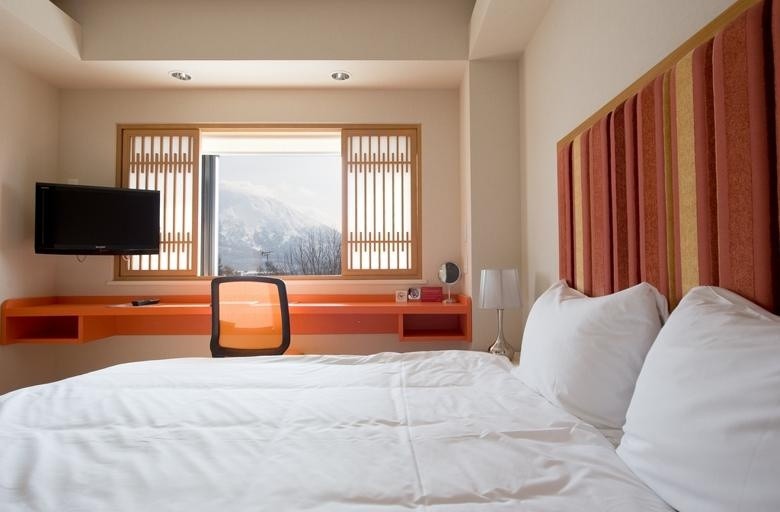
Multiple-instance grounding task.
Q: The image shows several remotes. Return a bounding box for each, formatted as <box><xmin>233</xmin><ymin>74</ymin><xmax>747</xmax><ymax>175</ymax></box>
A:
<box><xmin>132</xmin><ymin>297</ymin><xmax>160</xmax><ymax>306</ymax></box>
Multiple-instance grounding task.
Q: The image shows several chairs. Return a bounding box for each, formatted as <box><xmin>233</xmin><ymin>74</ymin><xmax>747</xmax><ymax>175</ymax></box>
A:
<box><xmin>206</xmin><ymin>275</ymin><xmax>292</xmax><ymax>354</ymax></box>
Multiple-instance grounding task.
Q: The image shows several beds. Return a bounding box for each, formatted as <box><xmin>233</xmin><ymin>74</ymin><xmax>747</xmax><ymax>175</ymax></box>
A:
<box><xmin>1</xmin><ymin>349</ymin><xmax>779</xmax><ymax>512</ymax></box>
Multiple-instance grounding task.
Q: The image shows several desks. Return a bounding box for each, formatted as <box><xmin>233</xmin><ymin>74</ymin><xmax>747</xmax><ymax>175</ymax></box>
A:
<box><xmin>1</xmin><ymin>293</ymin><xmax>471</xmax><ymax>344</ymax></box>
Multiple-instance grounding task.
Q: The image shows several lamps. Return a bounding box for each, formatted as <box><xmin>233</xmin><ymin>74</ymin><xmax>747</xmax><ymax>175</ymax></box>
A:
<box><xmin>478</xmin><ymin>266</ymin><xmax>526</xmax><ymax>359</ymax></box>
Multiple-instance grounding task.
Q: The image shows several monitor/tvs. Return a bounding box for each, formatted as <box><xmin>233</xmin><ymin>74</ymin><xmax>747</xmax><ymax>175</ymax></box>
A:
<box><xmin>35</xmin><ymin>182</ymin><xmax>160</xmax><ymax>255</ymax></box>
